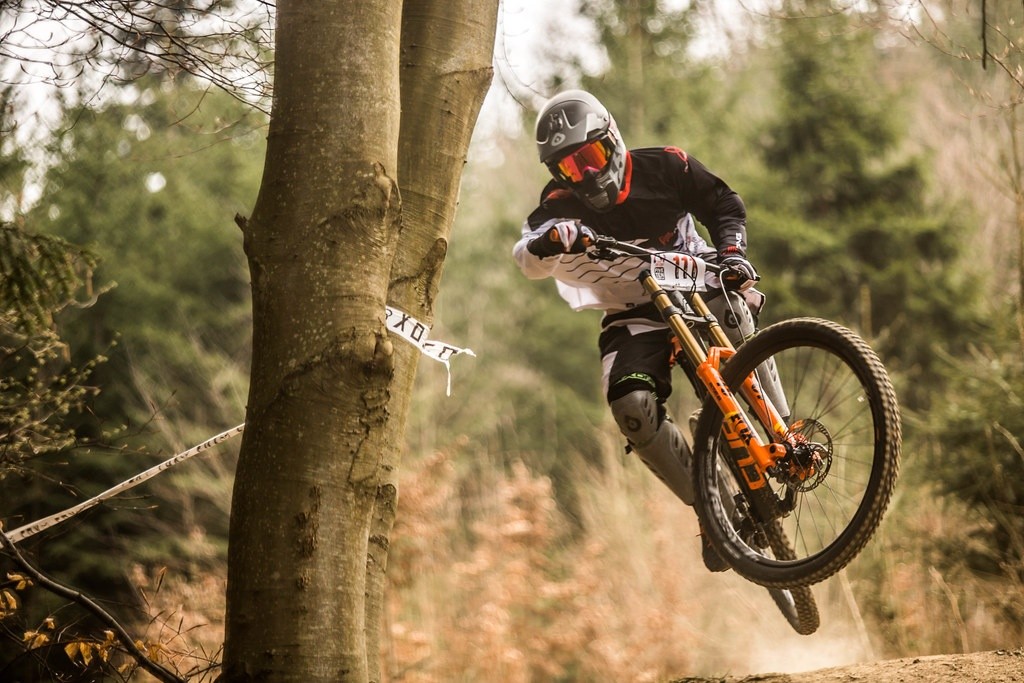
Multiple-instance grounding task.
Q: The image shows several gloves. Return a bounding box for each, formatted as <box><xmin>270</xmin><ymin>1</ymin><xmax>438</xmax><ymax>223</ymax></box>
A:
<box><xmin>717</xmin><ymin>249</ymin><xmax>758</xmax><ymax>292</ymax></box>
<box><xmin>534</xmin><ymin>219</ymin><xmax>598</xmax><ymax>259</ymax></box>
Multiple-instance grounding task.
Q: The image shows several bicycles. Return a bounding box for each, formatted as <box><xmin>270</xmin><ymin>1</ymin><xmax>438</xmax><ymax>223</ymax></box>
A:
<box><xmin>545</xmin><ymin>220</ymin><xmax>901</xmax><ymax>635</ymax></box>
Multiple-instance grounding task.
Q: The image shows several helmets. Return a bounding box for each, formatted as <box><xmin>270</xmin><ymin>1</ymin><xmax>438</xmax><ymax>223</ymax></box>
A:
<box><xmin>534</xmin><ymin>89</ymin><xmax>625</xmax><ymax>213</ymax></box>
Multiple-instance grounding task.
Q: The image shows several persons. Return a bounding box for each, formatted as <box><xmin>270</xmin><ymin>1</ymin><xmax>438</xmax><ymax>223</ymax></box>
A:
<box><xmin>509</xmin><ymin>88</ymin><xmax>802</xmax><ymax>573</ymax></box>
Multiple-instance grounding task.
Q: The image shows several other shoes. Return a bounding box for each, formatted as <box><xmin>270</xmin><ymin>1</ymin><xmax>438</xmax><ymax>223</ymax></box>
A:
<box><xmin>701</xmin><ymin>511</ymin><xmax>732</xmax><ymax>573</ymax></box>
